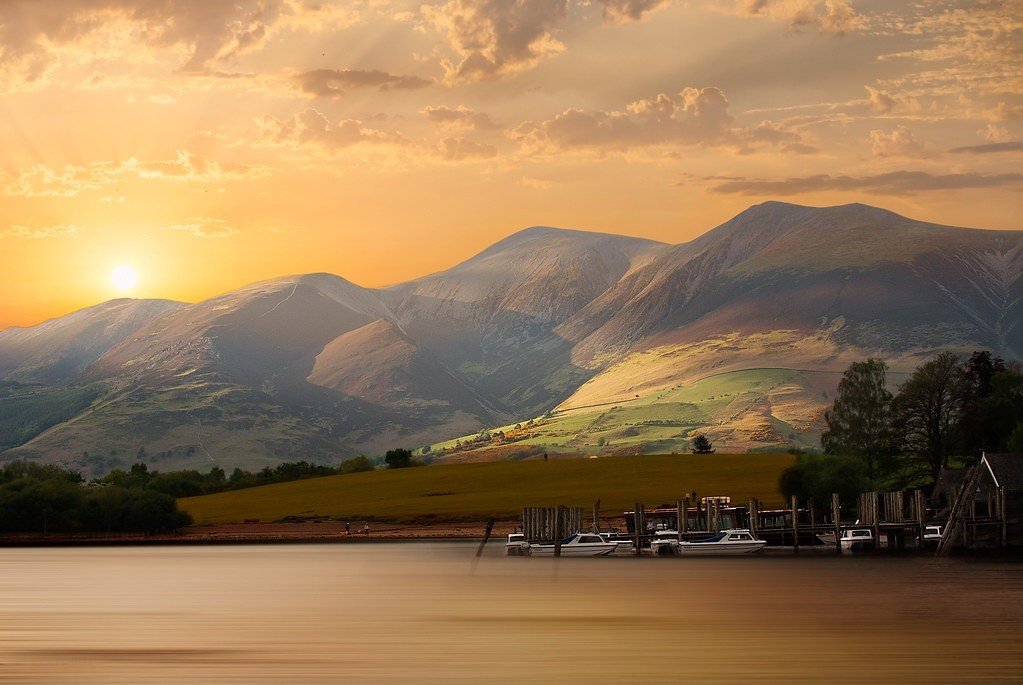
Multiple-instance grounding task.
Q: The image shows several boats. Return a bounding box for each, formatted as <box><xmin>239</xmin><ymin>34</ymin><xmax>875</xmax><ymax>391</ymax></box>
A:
<box><xmin>669</xmin><ymin>528</ymin><xmax>769</xmax><ymax>557</ymax></box>
<box><xmin>506</xmin><ymin>531</ymin><xmax>525</xmax><ymax>549</ymax></box>
<box><xmin>816</xmin><ymin>532</ymin><xmax>838</xmax><ymax>545</ymax></box>
<box><xmin>528</xmin><ymin>534</ymin><xmax>619</xmax><ymax>556</ymax></box>
<box><xmin>837</xmin><ymin>530</ymin><xmax>875</xmax><ymax>552</ymax></box>
<box><xmin>915</xmin><ymin>524</ymin><xmax>943</xmax><ymax>542</ymax></box>
